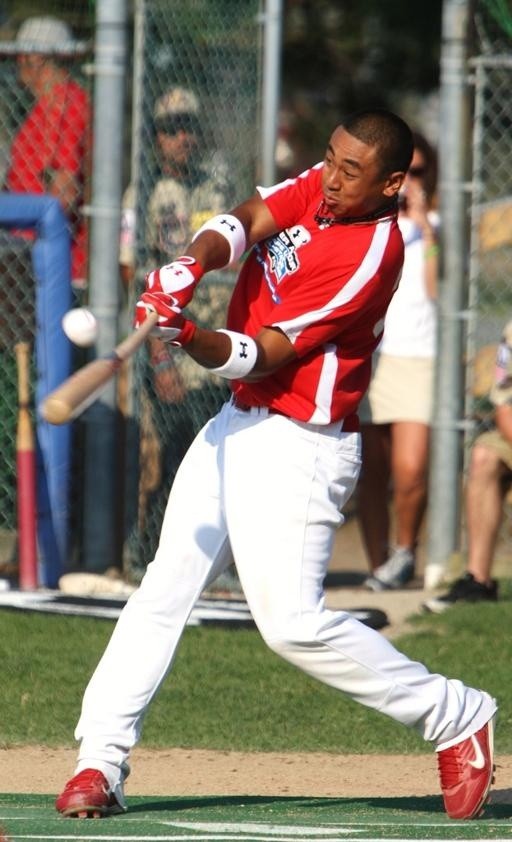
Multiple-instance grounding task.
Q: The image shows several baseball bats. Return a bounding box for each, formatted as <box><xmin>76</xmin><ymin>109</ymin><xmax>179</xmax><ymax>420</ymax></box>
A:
<box><xmin>40</xmin><ymin>311</ymin><xmax>158</xmax><ymax>424</ymax></box>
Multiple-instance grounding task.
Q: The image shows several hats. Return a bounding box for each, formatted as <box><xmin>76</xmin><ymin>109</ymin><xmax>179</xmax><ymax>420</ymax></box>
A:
<box><xmin>15</xmin><ymin>16</ymin><xmax>70</xmax><ymax>42</ymax></box>
<box><xmin>152</xmin><ymin>88</ymin><xmax>203</xmax><ymax>139</ymax></box>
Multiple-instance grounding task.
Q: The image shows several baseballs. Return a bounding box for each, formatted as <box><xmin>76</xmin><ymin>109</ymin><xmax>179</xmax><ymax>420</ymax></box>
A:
<box><xmin>62</xmin><ymin>307</ymin><xmax>98</xmax><ymax>346</ymax></box>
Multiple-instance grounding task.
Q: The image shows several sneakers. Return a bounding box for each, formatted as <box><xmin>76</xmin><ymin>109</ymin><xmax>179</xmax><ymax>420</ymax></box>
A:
<box><xmin>361</xmin><ymin>552</ymin><xmax>501</xmax><ymax>616</ymax></box>
<box><xmin>56</xmin><ymin>768</ymin><xmax>118</xmax><ymax>818</ymax></box>
<box><xmin>438</xmin><ymin>717</ymin><xmax>496</xmax><ymax>820</ymax></box>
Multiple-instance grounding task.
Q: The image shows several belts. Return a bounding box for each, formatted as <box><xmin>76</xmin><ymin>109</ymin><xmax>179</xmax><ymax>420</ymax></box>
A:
<box><xmin>232</xmin><ymin>399</ymin><xmax>360</xmax><ymax>433</ymax></box>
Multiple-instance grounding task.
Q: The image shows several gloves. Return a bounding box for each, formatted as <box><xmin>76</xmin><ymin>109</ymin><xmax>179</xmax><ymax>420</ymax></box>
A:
<box><xmin>133</xmin><ymin>292</ymin><xmax>196</xmax><ymax>348</ymax></box>
<box><xmin>145</xmin><ymin>256</ymin><xmax>206</xmax><ymax>308</ymax></box>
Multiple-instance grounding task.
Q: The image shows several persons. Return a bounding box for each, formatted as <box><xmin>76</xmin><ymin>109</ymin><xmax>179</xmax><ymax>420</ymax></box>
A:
<box><xmin>353</xmin><ymin>130</ymin><xmax>442</xmax><ymax>593</ymax></box>
<box><xmin>56</xmin><ymin>108</ymin><xmax>498</xmax><ymax>820</ymax></box>
<box><xmin>0</xmin><ymin>16</ymin><xmax>93</xmax><ymax>584</ymax></box>
<box><xmin>117</xmin><ymin>87</ymin><xmax>236</xmax><ymax>585</ymax></box>
<box><xmin>418</xmin><ymin>320</ymin><xmax>512</xmax><ymax>615</ymax></box>
